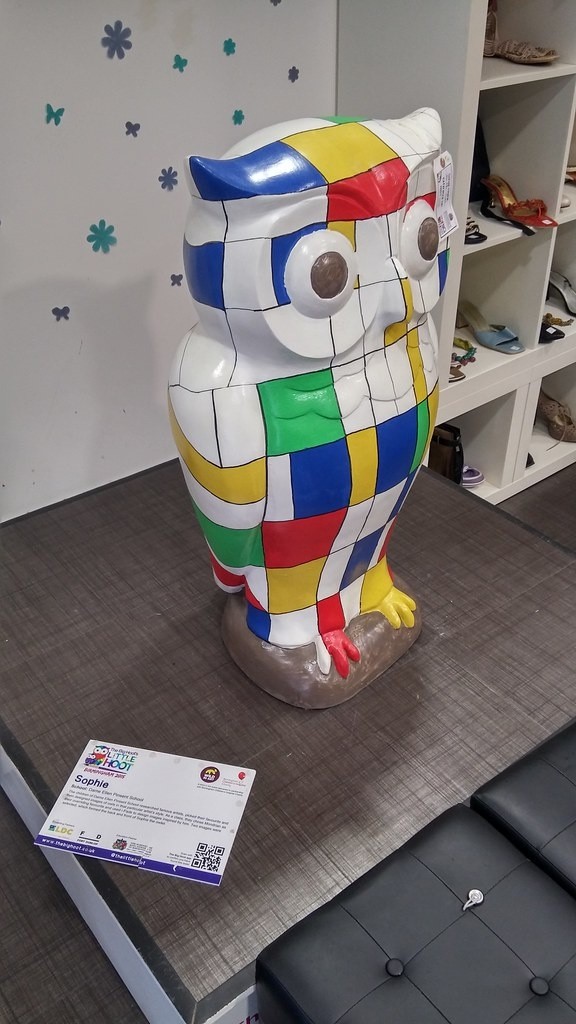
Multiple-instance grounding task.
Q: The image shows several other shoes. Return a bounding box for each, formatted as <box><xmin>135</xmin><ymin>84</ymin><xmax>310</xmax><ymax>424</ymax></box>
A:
<box><xmin>465</xmin><ymin>216</ymin><xmax>487</xmax><ymax>243</ymax></box>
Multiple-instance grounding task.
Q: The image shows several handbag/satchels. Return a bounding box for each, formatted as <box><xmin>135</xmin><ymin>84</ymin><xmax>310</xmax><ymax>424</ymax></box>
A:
<box><xmin>428</xmin><ymin>424</ymin><xmax>464</xmax><ymax>485</ymax></box>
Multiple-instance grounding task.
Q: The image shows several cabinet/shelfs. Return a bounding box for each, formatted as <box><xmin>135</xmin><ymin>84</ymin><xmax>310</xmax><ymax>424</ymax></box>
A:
<box><xmin>335</xmin><ymin>0</ymin><xmax>575</xmax><ymax>506</ymax></box>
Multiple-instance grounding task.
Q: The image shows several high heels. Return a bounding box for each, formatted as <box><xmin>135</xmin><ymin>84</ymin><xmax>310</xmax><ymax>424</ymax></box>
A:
<box><xmin>458</xmin><ymin>299</ymin><xmax>524</xmax><ymax>355</ymax></box>
<box><xmin>546</xmin><ymin>270</ymin><xmax>576</xmax><ymax>316</ymax></box>
<box><xmin>534</xmin><ymin>389</ymin><xmax>576</xmax><ymax>444</ymax></box>
<box><xmin>480</xmin><ymin>175</ymin><xmax>559</xmax><ymax>227</ymax></box>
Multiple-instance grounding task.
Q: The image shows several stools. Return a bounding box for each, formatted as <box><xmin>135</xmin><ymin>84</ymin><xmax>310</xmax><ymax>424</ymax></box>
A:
<box><xmin>255</xmin><ymin>804</ymin><xmax>576</xmax><ymax>1024</ymax></box>
<box><xmin>469</xmin><ymin>722</ymin><xmax>576</xmax><ymax>899</ymax></box>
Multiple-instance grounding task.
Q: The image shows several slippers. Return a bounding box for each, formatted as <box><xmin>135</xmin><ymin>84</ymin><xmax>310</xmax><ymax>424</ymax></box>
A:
<box><xmin>482</xmin><ymin>4</ymin><xmax>497</xmax><ymax>56</ymax></box>
<box><xmin>493</xmin><ymin>36</ymin><xmax>559</xmax><ymax>62</ymax></box>
